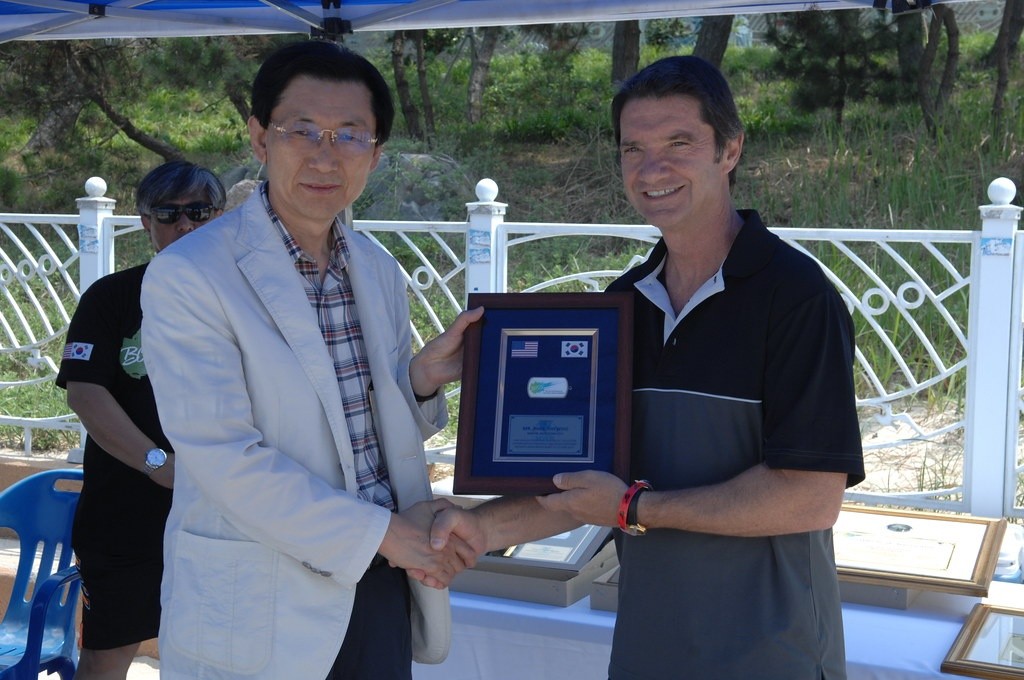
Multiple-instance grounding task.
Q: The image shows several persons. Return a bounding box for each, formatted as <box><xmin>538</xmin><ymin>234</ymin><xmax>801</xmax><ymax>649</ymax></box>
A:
<box><xmin>56</xmin><ymin>163</ymin><xmax>225</xmax><ymax>680</ymax></box>
<box><xmin>140</xmin><ymin>39</ymin><xmax>482</xmax><ymax>680</ymax></box>
<box><xmin>392</xmin><ymin>61</ymin><xmax>868</xmax><ymax>680</ymax></box>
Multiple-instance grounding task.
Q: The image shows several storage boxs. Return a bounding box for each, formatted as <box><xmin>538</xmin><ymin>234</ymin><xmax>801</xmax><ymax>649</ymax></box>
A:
<box><xmin>590</xmin><ymin>564</ymin><xmax>622</xmax><ymax>613</ymax></box>
<box><xmin>448</xmin><ymin>540</ymin><xmax>619</xmax><ymax>608</ymax></box>
<box><xmin>839</xmin><ymin>574</ymin><xmax>924</xmax><ymax>610</ymax></box>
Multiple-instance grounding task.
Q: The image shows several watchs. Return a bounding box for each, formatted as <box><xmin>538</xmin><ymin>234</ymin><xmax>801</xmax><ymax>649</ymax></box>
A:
<box><xmin>626</xmin><ymin>485</ymin><xmax>649</xmax><ymax>538</ymax></box>
<box><xmin>144</xmin><ymin>449</ymin><xmax>168</xmax><ymax>476</ymax></box>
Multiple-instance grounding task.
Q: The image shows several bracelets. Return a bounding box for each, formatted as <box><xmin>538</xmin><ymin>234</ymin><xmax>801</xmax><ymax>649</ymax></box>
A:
<box><xmin>416</xmin><ymin>389</ymin><xmax>442</xmax><ymax>404</ymax></box>
<box><xmin>616</xmin><ymin>480</ymin><xmax>650</xmax><ymax>533</ymax></box>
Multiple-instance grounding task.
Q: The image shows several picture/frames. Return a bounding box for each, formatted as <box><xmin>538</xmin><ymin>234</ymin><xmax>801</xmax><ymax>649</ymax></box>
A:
<box><xmin>453</xmin><ymin>291</ymin><xmax>633</xmax><ymax>497</ymax></box>
<box><xmin>479</xmin><ymin>522</ymin><xmax>612</xmax><ymax>570</ymax></box>
<box><xmin>832</xmin><ymin>503</ymin><xmax>1009</xmax><ymax>598</ymax></box>
<box><xmin>939</xmin><ymin>602</ymin><xmax>1024</xmax><ymax>680</ymax></box>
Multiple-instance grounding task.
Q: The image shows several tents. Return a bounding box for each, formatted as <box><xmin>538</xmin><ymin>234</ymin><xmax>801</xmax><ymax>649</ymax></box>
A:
<box><xmin>0</xmin><ymin>0</ymin><xmax>977</xmax><ymax>43</ymax></box>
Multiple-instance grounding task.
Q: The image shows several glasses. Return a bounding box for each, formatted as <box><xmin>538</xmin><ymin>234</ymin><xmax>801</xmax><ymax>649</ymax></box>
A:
<box><xmin>267</xmin><ymin>120</ymin><xmax>380</xmax><ymax>157</ymax></box>
<box><xmin>152</xmin><ymin>203</ymin><xmax>213</xmax><ymax>225</ymax></box>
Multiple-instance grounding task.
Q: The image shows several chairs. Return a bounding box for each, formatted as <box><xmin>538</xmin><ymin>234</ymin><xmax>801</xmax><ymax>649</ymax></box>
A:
<box><xmin>0</xmin><ymin>469</ymin><xmax>84</xmax><ymax>680</ymax></box>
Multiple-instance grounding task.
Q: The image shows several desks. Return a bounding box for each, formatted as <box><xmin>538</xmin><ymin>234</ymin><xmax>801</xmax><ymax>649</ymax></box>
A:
<box><xmin>411</xmin><ymin>581</ymin><xmax>1024</xmax><ymax>680</ymax></box>
